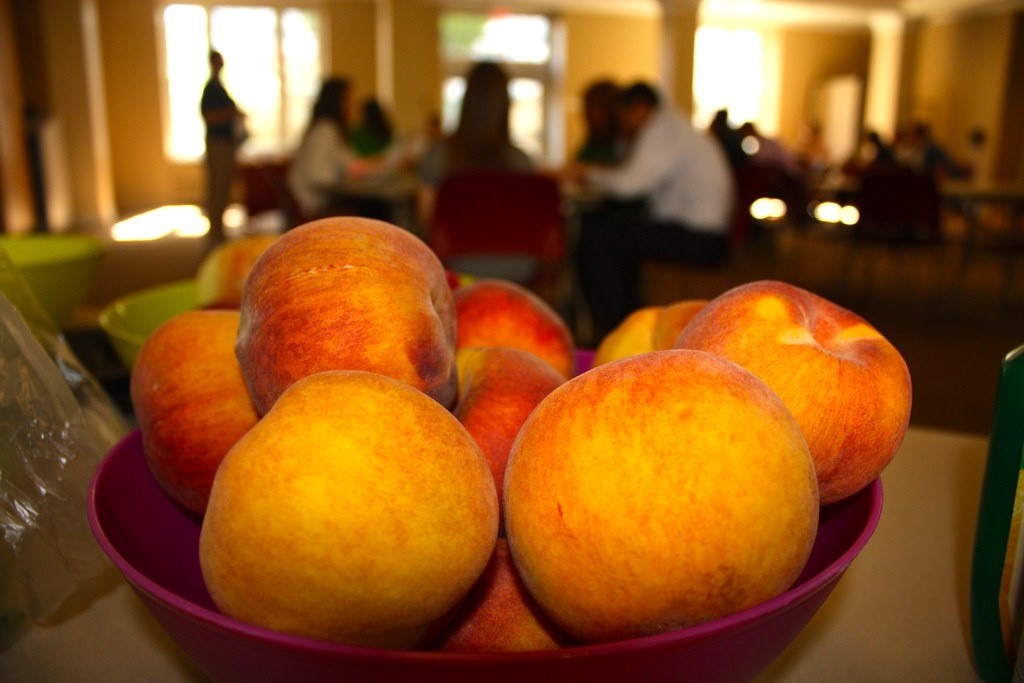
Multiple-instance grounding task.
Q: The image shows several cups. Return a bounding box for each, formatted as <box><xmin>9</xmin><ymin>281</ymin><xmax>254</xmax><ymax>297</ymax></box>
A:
<box><xmin>0</xmin><ymin>242</ymin><xmax>128</xmax><ymax>627</ymax></box>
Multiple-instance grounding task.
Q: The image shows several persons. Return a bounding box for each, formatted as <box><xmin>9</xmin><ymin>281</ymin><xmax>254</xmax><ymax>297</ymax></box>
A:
<box><xmin>413</xmin><ymin>62</ymin><xmax>562</xmax><ymax>299</ymax></box>
<box><xmin>568</xmin><ymin>82</ymin><xmax>738</xmax><ymax>344</ymax></box>
<box><xmin>198</xmin><ymin>52</ymin><xmax>248</xmax><ymax>246</ymax></box>
<box><xmin>541</xmin><ymin>80</ymin><xmax>651</xmax><ymax>246</ymax></box>
<box><xmin>700</xmin><ymin>105</ymin><xmax>980</xmax><ymax>253</ymax></box>
<box><xmin>347</xmin><ymin>93</ymin><xmax>409</xmax><ymax>218</ymax></box>
<box><xmin>282</xmin><ymin>75</ymin><xmax>433</xmax><ymax>219</ymax></box>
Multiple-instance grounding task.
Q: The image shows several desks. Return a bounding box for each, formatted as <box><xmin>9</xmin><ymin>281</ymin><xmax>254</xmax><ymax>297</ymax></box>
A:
<box><xmin>2</xmin><ymin>424</ymin><xmax>990</xmax><ymax>682</ymax></box>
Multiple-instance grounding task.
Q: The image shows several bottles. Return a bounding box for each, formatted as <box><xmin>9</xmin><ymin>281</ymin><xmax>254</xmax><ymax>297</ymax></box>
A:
<box><xmin>969</xmin><ymin>342</ymin><xmax>1024</xmax><ymax>683</ymax></box>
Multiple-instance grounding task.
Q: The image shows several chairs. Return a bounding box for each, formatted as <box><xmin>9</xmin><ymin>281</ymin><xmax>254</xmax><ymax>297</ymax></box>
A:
<box><xmin>428</xmin><ymin>170</ymin><xmax>568</xmax><ymax>327</ymax></box>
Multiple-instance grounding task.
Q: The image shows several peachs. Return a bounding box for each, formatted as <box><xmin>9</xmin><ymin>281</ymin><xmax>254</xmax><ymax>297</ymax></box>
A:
<box><xmin>130</xmin><ymin>216</ymin><xmax>913</xmax><ymax>654</ymax></box>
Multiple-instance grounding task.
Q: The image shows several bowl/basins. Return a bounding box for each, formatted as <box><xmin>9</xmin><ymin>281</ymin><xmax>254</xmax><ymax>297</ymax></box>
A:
<box><xmin>0</xmin><ymin>233</ymin><xmax>106</xmax><ymax>330</ymax></box>
<box><xmin>96</xmin><ymin>280</ymin><xmax>197</xmax><ymax>371</ymax></box>
<box><xmin>88</xmin><ymin>347</ymin><xmax>884</xmax><ymax>683</ymax></box>
